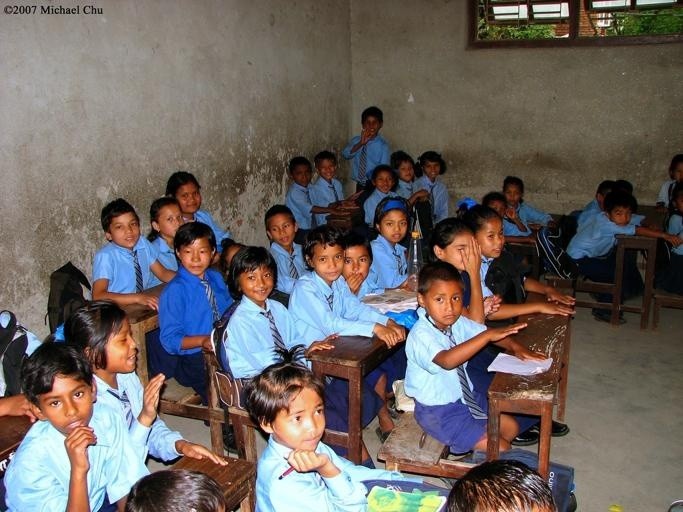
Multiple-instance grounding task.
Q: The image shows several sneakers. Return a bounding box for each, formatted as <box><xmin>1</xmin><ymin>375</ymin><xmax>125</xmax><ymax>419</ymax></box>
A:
<box><xmin>386</xmin><ymin>396</ymin><xmax>405</xmax><ymax>415</ymax></box>
<box><xmin>220</xmin><ymin>424</ymin><xmax>239</xmax><ymax>454</ymax></box>
<box><xmin>375</xmin><ymin>427</ymin><xmax>392</xmax><ymax>445</ymax></box>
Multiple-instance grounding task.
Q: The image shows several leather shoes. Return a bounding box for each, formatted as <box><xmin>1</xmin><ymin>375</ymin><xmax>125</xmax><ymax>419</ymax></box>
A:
<box><xmin>531</xmin><ymin>418</ymin><xmax>571</xmax><ymax>438</ymax></box>
<box><xmin>591</xmin><ymin>307</ymin><xmax>627</xmax><ymax>325</ymax></box>
<box><xmin>511</xmin><ymin>431</ymin><xmax>540</xmax><ymax>446</ymax></box>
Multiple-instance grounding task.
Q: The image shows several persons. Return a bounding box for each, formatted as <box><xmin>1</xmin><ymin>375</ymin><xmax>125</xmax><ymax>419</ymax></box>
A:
<box><xmin>0</xmin><ymin>106</ymin><xmax>683</xmax><ymax>512</ymax></box>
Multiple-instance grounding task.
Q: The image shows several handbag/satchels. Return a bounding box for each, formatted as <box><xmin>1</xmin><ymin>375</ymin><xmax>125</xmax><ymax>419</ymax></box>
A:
<box><xmin>472</xmin><ymin>448</ymin><xmax>575</xmax><ymax>512</ymax></box>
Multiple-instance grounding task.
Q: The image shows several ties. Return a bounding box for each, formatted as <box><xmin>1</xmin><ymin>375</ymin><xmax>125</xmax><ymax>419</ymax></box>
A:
<box><xmin>199</xmin><ymin>279</ymin><xmax>221</xmax><ymax>323</ymax></box>
<box><xmin>406</xmin><ymin>184</ymin><xmax>414</xmax><ymax>196</ymax></box>
<box><xmin>428</xmin><ymin>184</ymin><xmax>436</xmax><ymax>219</ymax></box>
<box><xmin>441</xmin><ymin>325</ymin><xmax>489</xmax><ymax>420</ymax></box>
<box><xmin>326</xmin><ymin>294</ymin><xmax>335</xmax><ymax>312</ymax></box>
<box><xmin>301</xmin><ymin>190</ymin><xmax>318</xmax><ymax>229</ymax></box>
<box><xmin>129</xmin><ymin>250</ymin><xmax>143</xmax><ymax>294</ymax></box>
<box><xmin>259</xmin><ymin>310</ymin><xmax>287</xmax><ymax>354</ymax></box>
<box><xmin>286</xmin><ymin>255</ymin><xmax>300</xmax><ymax>280</ymax></box>
<box><xmin>328</xmin><ymin>184</ymin><xmax>339</xmax><ymax>201</ymax></box>
<box><xmin>393</xmin><ymin>253</ymin><xmax>404</xmax><ymax>277</ymax></box>
<box><xmin>357</xmin><ymin>144</ymin><xmax>367</xmax><ymax>181</ymax></box>
<box><xmin>107</xmin><ymin>390</ymin><xmax>136</xmax><ymax>435</ymax></box>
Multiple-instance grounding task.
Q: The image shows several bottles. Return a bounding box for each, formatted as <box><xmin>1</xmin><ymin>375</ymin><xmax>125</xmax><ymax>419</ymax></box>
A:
<box><xmin>406</xmin><ymin>231</ymin><xmax>421</xmax><ymax>289</ymax></box>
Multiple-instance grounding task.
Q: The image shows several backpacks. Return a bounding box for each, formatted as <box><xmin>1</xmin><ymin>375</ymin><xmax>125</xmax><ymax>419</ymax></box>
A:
<box><xmin>534</xmin><ymin>225</ymin><xmax>577</xmax><ymax>281</ymax></box>
<box><xmin>485</xmin><ymin>253</ymin><xmax>528</xmax><ymax>325</ymax></box>
<box><xmin>46</xmin><ymin>262</ymin><xmax>90</xmax><ymax>336</ymax></box>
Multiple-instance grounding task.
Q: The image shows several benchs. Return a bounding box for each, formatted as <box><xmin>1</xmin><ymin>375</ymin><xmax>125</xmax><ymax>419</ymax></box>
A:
<box><xmin>230</xmin><ymin>406</ymin><xmax>261</xmax><ymax>459</ymax></box>
<box><xmin>159</xmin><ymin>376</ymin><xmax>209</xmax><ymax>421</ymax></box>
<box><xmin>653</xmin><ymin>290</ymin><xmax>683</xmax><ymax>330</ymax></box>
<box><xmin>377</xmin><ymin>411</ymin><xmax>476</xmax><ymax>477</ymax></box>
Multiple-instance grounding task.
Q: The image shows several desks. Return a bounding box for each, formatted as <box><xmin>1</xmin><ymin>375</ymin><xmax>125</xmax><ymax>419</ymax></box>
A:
<box><xmin>487</xmin><ymin>289</ymin><xmax>573</xmax><ymax>482</ymax></box>
<box><xmin>121</xmin><ymin>281</ymin><xmax>167</xmax><ymax>389</ymax></box>
<box><xmin>203</xmin><ymin>337</ymin><xmax>224</xmax><ymax>455</ymax></box>
<box><xmin>507</xmin><ymin>214</ymin><xmax>560</xmax><ymax>280</ymax></box>
<box><xmin>326</xmin><ymin>210</ymin><xmax>362</xmax><ymax>228</ymax></box>
<box><xmin>611</xmin><ymin>206</ymin><xmax>657</xmax><ymax>330</ymax></box>
<box><xmin>171</xmin><ymin>455</ymin><xmax>257</xmax><ymax>511</ymax></box>
<box><xmin>0</xmin><ymin>416</ymin><xmax>33</xmax><ymax>461</ymax></box>
<box><xmin>309</xmin><ymin>336</ymin><xmax>405</xmax><ymax>466</ymax></box>
